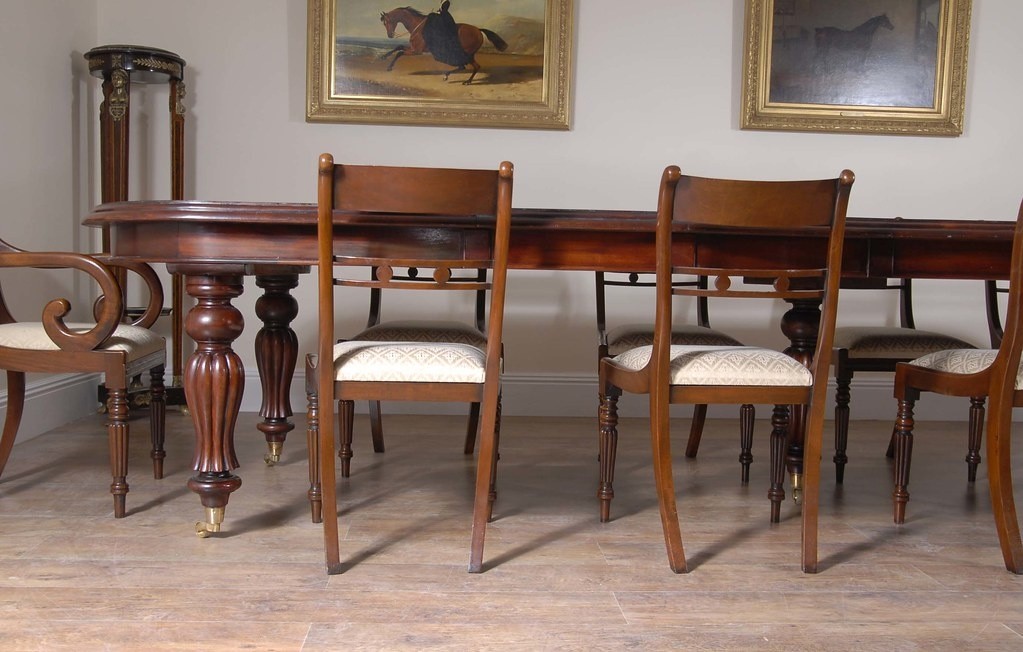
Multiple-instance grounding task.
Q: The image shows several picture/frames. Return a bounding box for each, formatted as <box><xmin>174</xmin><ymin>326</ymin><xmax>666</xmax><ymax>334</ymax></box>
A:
<box><xmin>305</xmin><ymin>0</ymin><xmax>573</xmax><ymax>131</ymax></box>
<box><xmin>739</xmin><ymin>0</ymin><xmax>973</xmax><ymax>136</ymax></box>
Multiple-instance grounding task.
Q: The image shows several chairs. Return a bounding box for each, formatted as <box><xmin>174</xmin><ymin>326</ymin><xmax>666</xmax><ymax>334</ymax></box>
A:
<box><xmin>305</xmin><ymin>152</ymin><xmax>514</xmax><ymax>578</ymax></box>
<box><xmin>597</xmin><ymin>166</ymin><xmax>854</xmax><ymax>576</ymax></box>
<box><xmin>810</xmin><ymin>218</ymin><xmax>987</xmax><ymax>481</ymax></box>
<box><xmin>0</xmin><ymin>238</ymin><xmax>167</xmax><ymax>520</ymax></box>
<box><xmin>893</xmin><ymin>200</ymin><xmax>1023</xmax><ymax>574</ymax></box>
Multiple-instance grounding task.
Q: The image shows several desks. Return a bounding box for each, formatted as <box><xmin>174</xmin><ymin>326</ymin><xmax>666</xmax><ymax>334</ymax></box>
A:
<box><xmin>83</xmin><ymin>200</ymin><xmax>1019</xmax><ymax>541</ymax></box>
